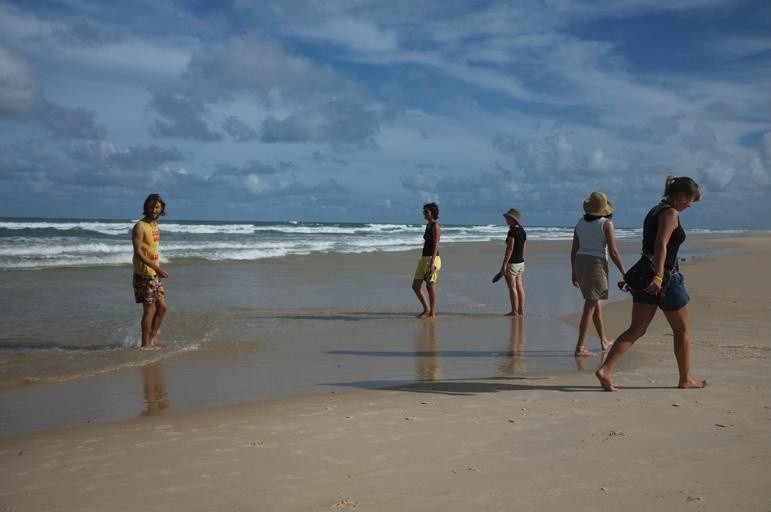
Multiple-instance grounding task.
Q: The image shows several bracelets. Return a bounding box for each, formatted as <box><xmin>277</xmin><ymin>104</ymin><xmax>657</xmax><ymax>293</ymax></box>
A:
<box><xmin>653</xmin><ymin>274</ymin><xmax>663</xmax><ymax>282</ymax></box>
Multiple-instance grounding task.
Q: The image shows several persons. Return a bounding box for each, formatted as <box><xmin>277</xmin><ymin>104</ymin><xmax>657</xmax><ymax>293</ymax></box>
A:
<box><xmin>593</xmin><ymin>173</ymin><xmax>708</xmax><ymax>392</ymax></box>
<box><xmin>411</xmin><ymin>201</ymin><xmax>444</xmax><ymax>320</ymax></box>
<box><xmin>129</xmin><ymin>192</ymin><xmax>170</xmax><ymax>348</ymax></box>
<box><xmin>499</xmin><ymin>208</ymin><xmax>528</xmax><ymax>317</ymax></box>
<box><xmin>569</xmin><ymin>191</ymin><xmax>627</xmax><ymax>357</ymax></box>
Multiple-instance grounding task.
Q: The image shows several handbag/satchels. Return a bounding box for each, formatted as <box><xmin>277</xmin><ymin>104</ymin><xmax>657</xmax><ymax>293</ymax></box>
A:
<box><xmin>623</xmin><ymin>255</ymin><xmax>673</xmax><ymax>302</ymax></box>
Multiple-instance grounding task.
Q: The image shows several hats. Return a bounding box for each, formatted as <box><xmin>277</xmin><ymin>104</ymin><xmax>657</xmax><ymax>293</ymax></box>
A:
<box><xmin>502</xmin><ymin>208</ymin><xmax>520</xmax><ymax>225</ymax></box>
<box><xmin>583</xmin><ymin>192</ymin><xmax>613</xmax><ymax>216</ymax></box>
